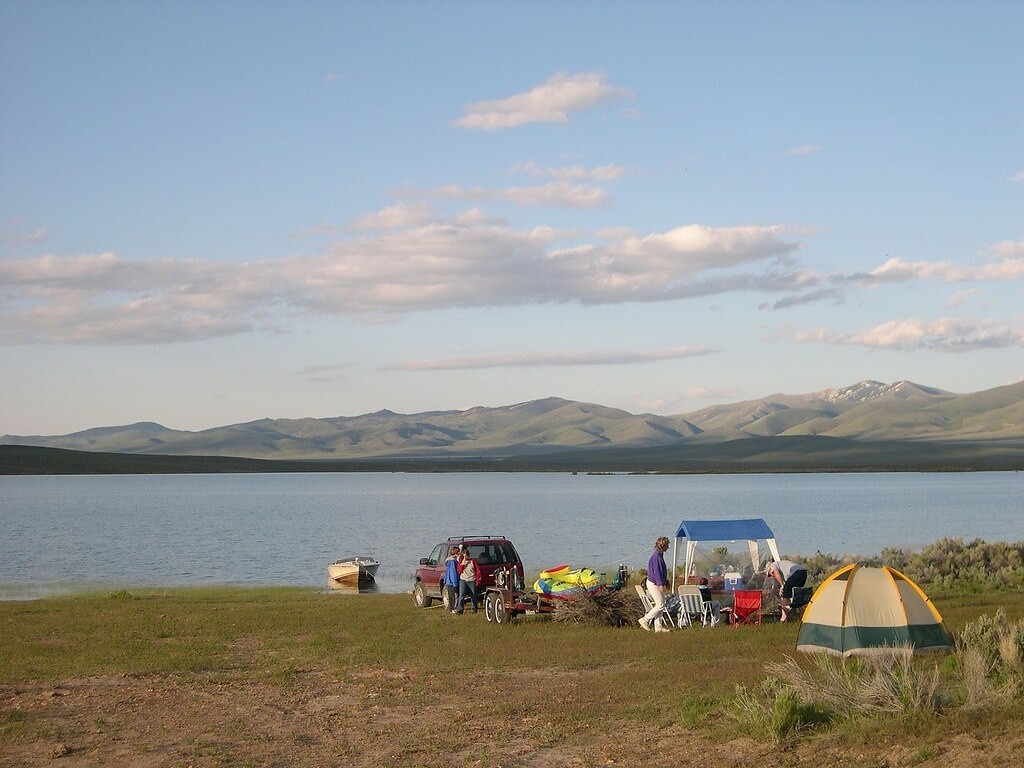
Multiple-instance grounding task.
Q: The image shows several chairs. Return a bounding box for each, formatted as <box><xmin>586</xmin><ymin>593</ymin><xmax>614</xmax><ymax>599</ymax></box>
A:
<box><xmin>731</xmin><ymin>590</ymin><xmax>763</xmax><ymax>628</ymax></box>
<box><xmin>677</xmin><ymin>585</ymin><xmax>716</xmax><ymax>629</ymax></box>
<box><xmin>746</xmin><ymin>570</ymin><xmax>767</xmax><ymax>588</ymax></box>
<box><xmin>478</xmin><ymin>552</ymin><xmax>491</xmax><ymax>561</ymax></box>
<box><xmin>779</xmin><ymin>586</ymin><xmax>813</xmax><ymax>623</ymax></box>
<box><xmin>634</xmin><ymin>585</ymin><xmax>680</xmax><ymax>627</ymax></box>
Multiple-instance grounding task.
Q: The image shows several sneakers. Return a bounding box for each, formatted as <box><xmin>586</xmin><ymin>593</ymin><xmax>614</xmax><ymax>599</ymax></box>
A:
<box><xmin>638</xmin><ymin>618</ymin><xmax>651</xmax><ymax>632</ymax></box>
<box><xmin>452</xmin><ymin>610</ymin><xmax>460</xmax><ymax>616</ymax></box>
<box><xmin>654</xmin><ymin>626</ymin><xmax>670</xmax><ymax>633</ymax></box>
<box><xmin>703</xmin><ymin>621</ymin><xmax>708</xmax><ymax>625</ymax></box>
<box><xmin>711</xmin><ymin>623</ymin><xmax>720</xmax><ymax>627</ymax></box>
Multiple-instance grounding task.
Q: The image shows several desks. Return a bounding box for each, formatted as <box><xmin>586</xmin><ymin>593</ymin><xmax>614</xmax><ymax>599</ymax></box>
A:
<box><xmin>679</xmin><ymin>576</ymin><xmax>723</xmax><ymax>590</ymax></box>
<box><xmin>710</xmin><ymin>589</ymin><xmax>779</xmax><ymax>616</ymax></box>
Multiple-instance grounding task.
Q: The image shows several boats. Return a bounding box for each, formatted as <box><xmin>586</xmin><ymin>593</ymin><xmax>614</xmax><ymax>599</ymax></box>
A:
<box><xmin>532</xmin><ymin>564</ymin><xmax>607</xmax><ymax>601</ymax></box>
<box><xmin>328</xmin><ymin>555</ymin><xmax>381</xmax><ymax>590</ymax></box>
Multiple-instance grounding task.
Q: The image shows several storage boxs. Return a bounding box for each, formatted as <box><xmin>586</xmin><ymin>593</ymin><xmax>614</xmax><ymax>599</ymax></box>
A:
<box><xmin>723</xmin><ymin>573</ymin><xmax>744</xmax><ymax>590</ymax></box>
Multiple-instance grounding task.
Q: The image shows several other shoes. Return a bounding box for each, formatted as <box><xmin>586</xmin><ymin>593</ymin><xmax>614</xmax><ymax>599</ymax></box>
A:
<box><xmin>780</xmin><ymin>616</ymin><xmax>788</xmax><ymax>625</ymax></box>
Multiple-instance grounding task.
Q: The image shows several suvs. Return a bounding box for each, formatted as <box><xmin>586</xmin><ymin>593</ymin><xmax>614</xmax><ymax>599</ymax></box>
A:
<box><xmin>414</xmin><ymin>536</ymin><xmax>525</xmax><ymax>607</ymax></box>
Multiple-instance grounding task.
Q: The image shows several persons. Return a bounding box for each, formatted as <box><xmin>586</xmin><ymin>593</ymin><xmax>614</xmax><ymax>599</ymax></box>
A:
<box><xmin>698</xmin><ymin>578</ymin><xmax>720</xmax><ymax>625</ymax></box>
<box><xmin>637</xmin><ymin>537</ymin><xmax>671</xmax><ymax>632</ymax></box>
<box><xmin>452</xmin><ymin>550</ymin><xmax>481</xmax><ymax>616</ymax></box>
<box><xmin>443</xmin><ymin>547</ymin><xmax>461</xmax><ymax>613</ymax></box>
<box><xmin>764</xmin><ymin>560</ymin><xmax>808</xmax><ymax>623</ymax></box>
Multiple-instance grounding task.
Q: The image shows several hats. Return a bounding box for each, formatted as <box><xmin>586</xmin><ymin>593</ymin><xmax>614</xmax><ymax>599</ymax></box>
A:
<box><xmin>765</xmin><ymin>561</ymin><xmax>772</xmax><ymax>577</ymax></box>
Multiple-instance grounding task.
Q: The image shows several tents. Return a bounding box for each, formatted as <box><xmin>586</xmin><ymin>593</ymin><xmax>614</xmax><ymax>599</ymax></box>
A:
<box><xmin>672</xmin><ymin>519</ymin><xmax>781</xmax><ymax>594</ymax></box>
<box><xmin>795</xmin><ymin>562</ymin><xmax>956</xmax><ymax>659</ymax></box>
